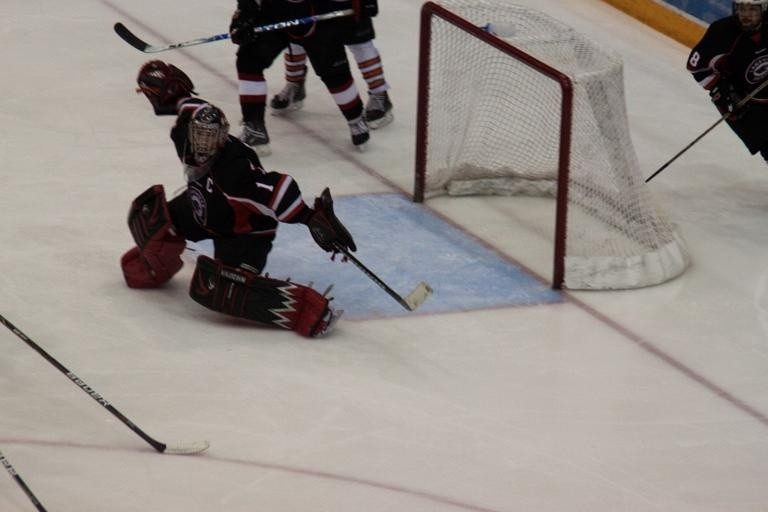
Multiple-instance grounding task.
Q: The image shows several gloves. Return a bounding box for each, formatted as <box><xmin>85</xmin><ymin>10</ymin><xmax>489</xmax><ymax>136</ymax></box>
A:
<box><xmin>230</xmin><ymin>0</ymin><xmax>259</xmax><ymax>45</ymax></box>
<box><xmin>308</xmin><ymin>188</ymin><xmax>356</xmax><ymax>252</ymax></box>
<box><xmin>138</xmin><ymin>60</ymin><xmax>193</xmax><ymax>115</ymax></box>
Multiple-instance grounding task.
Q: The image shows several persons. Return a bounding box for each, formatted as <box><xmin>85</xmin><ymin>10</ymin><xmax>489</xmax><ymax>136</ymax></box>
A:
<box><xmin>685</xmin><ymin>1</ymin><xmax>768</xmax><ymax>159</ymax></box>
<box><xmin>118</xmin><ymin>60</ymin><xmax>358</xmax><ymax>334</ymax></box>
<box><xmin>269</xmin><ymin>1</ymin><xmax>396</xmax><ymax>124</ymax></box>
<box><xmin>229</xmin><ymin>0</ymin><xmax>371</xmax><ymax>153</ymax></box>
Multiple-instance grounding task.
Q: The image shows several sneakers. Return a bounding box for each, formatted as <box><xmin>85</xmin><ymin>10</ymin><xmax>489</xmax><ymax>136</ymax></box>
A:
<box><xmin>271</xmin><ymin>83</ymin><xmax>305</xmax><ymax>108</ymax></box>
<box><xmin>238</xmin><ymin>120</ymin><xmax>268</xmax><ymax>145</ymax></box>
<box><xmin>348</xmin><ymin>91</ymin><xmax>392</xmax><ymax>145</ymax></box>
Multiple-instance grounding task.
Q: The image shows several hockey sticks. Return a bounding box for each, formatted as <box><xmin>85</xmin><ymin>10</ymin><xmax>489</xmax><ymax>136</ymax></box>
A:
<box><xmin>0</xmin><ymin>316</ymin><xmax>208</xmax><ymax>455</ymax></box>
<box><xmin>332</xmin><ymin>240</ymin><xmax>432</xmax><ymax>311</ymax></box>
<box><xmin>115</xmin><ymin>9</ymin><xmax>355</xmax><ymax>53</ymax></box>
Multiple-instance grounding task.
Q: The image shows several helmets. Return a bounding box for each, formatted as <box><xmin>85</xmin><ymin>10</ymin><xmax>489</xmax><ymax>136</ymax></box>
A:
<box><xmin>189</xmin><ymin>103</ymin><xmax>230</xmax><ymax>150</ymax></box>
